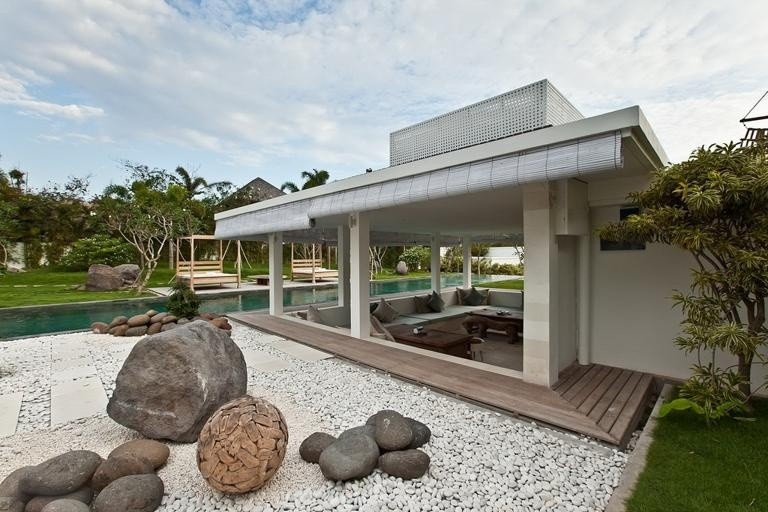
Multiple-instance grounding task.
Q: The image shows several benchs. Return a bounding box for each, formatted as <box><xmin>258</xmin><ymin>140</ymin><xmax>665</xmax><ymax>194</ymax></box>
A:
<box><xmin>383</xmin><ymin>287</ymin><xmax>470</xmax><ymax>327</ymax></box>
<box><xmin>177</xmin><ymin>260</ymin><xmax>240</xmax><ymax>293</ymax></box>
<box><xmin>292</xmin><ymin>259</ymin><xmax>339</xmax><ymax>283</ymax></box>
<box><xmin>470</xmin><ymin>288</ymin><xmax>523</xmax><ymax>312</ymax></box>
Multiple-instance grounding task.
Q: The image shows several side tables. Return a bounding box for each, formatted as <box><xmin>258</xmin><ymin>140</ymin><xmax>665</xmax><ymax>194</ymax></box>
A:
<box><xmin>247</xmin><ymin>274</ymin><xmax>288</xmax><ymax>286</ymax></box>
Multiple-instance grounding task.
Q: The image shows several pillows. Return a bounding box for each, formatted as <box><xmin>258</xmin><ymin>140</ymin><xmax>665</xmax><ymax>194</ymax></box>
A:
<box><xmin>369</xmin><ymin>296</ymin><xmax>398</xmax><ymax>324</ymax></box>
<box><xmin>292</xmin><ymin>304</ymin><xmax>327</xmax><ymax>324</ymax></box>
